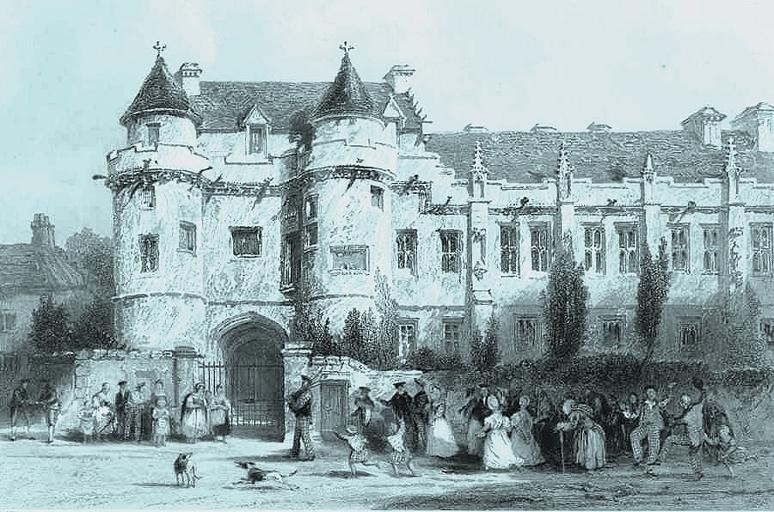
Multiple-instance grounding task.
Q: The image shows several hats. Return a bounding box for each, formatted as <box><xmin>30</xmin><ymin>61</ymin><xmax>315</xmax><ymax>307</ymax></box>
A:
<box><xmin>393</xmin><ymin>382</ymin><xmax>406</xmax><ymax>388</ymax></box>
<box><xmin>414</xmin><ymin>378</ymin><xmax>426</xmax><ymax>386</ymax></box>
<box><xmin>301</xmin><ymin>375</ymin><xmax>312</xmax><ymax>382</ymax></box>
<box><xmin>118</xmin><ymin>381</ymin><xmax>127</xmax><ymax>385</ymax></box>
<box><xmin>359</xmin><ymin>387</ymin><xmax>371</xmax><ymax>392</ymax></box>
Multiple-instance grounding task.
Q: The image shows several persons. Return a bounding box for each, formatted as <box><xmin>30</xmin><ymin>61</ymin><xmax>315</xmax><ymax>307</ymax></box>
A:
<box><xmin>8</xmin><ymin>379</ymin><xmax>39</xmax><ymax>440</ymax></box>
<box><xmin>79</xmin><ymin>381</ymin><xmax>232</xmax><ymax>447</ymax></box>
<box><xmin>282</xmin><ymin>375</ymin><xmax>315</xmax><ymax>461</ymax></box>
<box><xmin>34</xmin><ymin>385</ymin><xmax>63</xmax><ymax>443</ymax></box>
<box><xmin>332</xmin><ymin>377</ymin><xmax>737</xmax><ymax>480</ymax></box>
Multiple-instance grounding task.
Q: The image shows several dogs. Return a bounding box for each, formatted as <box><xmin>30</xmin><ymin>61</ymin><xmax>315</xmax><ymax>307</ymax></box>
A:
<box><xmin>231</xmin><ymin>460</ymin><xmax>299</xmax><ymax>491</ymax></box>
<box><xmin>173</xmin><ymin>452</ymin><xmax>199</xmax><ymax>487</ymax></box>
